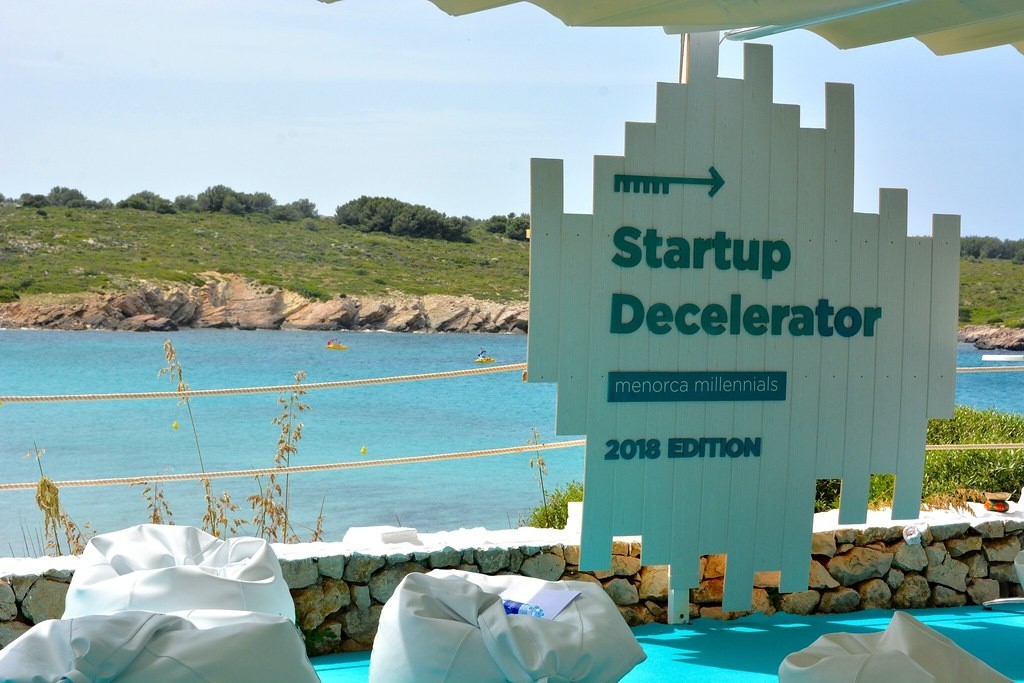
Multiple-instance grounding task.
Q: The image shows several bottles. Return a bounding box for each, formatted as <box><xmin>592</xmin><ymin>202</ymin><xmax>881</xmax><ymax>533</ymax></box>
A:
<box><xmin>502</xmin><ymin>599</ymin><xmax>544</xmax><ymax>618</ymax></box>
